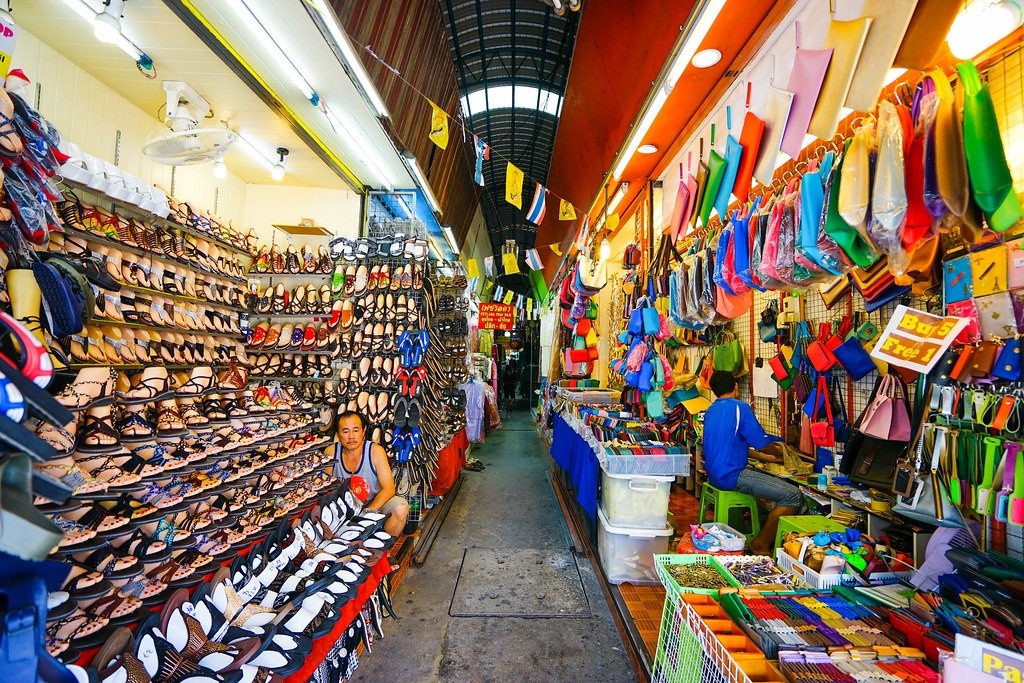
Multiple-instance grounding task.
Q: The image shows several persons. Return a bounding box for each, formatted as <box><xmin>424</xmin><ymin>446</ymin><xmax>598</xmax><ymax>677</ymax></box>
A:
<box><xmin>323</xmin><ymin>410</ymin><xmax>409</xmax><ymax>537</ymax></box>
<box><xmin>502</xmin><ymin>358</ymin><xmax>519</xmax><ymax>408</ymax></box>
<box><xmin>703</xmin><ymin>370</ymin><xmax>804</xmax><ymax>557</ymax></box>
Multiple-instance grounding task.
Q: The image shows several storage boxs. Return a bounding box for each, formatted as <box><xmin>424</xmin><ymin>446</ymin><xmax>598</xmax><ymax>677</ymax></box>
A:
<box><xmin>556</xmin><ymin>386</ymin><xmax>622</xmax><ymax>404</ymax></box>
<box><xmin>595</xmin><ymin>503</ymin><xmax>674</xmax><ymax>585</ymax></box>
<box><xmin>598</xmin><ymin>463</ymin><xmax>677</xmax><ymax>529</ymax></box>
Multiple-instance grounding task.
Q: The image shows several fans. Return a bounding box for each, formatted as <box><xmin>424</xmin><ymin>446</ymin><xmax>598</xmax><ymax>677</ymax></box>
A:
<box><xmin>143</xmin><ymin>81</ymin><xmax>234</xmax><ymax>161</ymax></box>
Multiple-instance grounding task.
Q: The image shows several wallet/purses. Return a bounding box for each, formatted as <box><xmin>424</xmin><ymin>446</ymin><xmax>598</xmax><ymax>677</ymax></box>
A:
<box><xmin>722</xmin><ymin>546</ymin><xmax>1024</xmax><ymax>683</ymax></box>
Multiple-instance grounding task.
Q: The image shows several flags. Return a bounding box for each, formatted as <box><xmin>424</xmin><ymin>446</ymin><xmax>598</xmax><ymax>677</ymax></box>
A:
<box><xmin>470</xmin><ymin>276</ymin><xmax>480</xmax><ymax>292</ymax></box>
<box><xmin>484</xmin><ymin>256</ymin><xmax>494</xmax><ymax>277</ymax></box>
<box><xmin>493</xmin><ymin>285</ymin><xmax>503</xmax><ymax>302</ymax></box>
<box><xmin>503</xmin><ymin>290</ymin><xmax>514</xmax><ymax>305</ymax></box>
<box><xmin>549</xmin><ymin>242</ymin><xmax>563</xmax><ymax>257</ymax></box>
<box><xmin>526</xmin><ymin>182</ymin><xmax>551</xmax><ymax>226</ymax></box>
<box><xmin>515</xmin><ymin>293</ymin><xmax>524</xmax><ymax>309</ymax></box>
<box><xmin>426</xmin><ymin>98</ymin><xmax>451</xmax><ymax>151</ymax></box>
<box><xmin>558</xmin><ymin>198</ymin><xmax>577</xmax><ymax>220</ymax></box>
<box><xmin>474</xmin><ymin>135</ymin><xmax>490</xmax><ymax>187</ymax></box>
<box><xmin>503</xmin><ymin>252</ymin><xmax>520</xmax><ymax>275</ymax></box>
<box><xmin>485</xmin><ymin>281</ymin><xmax>548</xmax><ymax>328</ymax></box>
<box><xmin>524</xmin><ymin>248</ymin><xmax>545</xmax><ymax>271</ymax></box>
<box><xmin>505</xmin><ymin>161</ymin><xmax>524</xmax><ymax>210</ymax></box>
<box><xmin>573</xmin><ymin>213</ymin><xmax>591</xmax><ymax>254</ymax></box>
<box><xmin>466</xmin><ymin>259</ymin><xmax>481</xmax><ymax>278</ymax></box>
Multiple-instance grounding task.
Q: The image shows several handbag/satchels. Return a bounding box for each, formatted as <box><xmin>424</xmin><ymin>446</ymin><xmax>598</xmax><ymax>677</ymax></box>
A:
<box><xmin>558</xmin><ymin>0</ymin><xmax>1023</xmax><ymax>535</ymax></box>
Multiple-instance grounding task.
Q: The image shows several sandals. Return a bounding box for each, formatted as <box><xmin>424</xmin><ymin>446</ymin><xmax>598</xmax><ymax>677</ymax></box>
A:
<box><xmin>0</xmin><ymin>84</ymin><xmax>471</xmax><ymax>683</ymax></box>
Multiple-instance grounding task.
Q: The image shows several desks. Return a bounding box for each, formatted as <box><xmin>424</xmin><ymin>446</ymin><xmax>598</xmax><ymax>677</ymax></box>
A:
<box><xmin>551</xmin><ymin>409</ymin><xmax>686</xmax><ymax>544</ymax></box>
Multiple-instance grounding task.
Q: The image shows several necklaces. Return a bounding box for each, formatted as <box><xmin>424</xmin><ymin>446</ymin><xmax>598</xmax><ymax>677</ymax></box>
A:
<box><xmin>347</xmin><ymin>463</ymin><xmax>357</xmax><ymax>476</ymax></box>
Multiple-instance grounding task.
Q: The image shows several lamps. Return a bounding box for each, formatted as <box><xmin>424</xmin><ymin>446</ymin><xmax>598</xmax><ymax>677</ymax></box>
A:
<box><xmin>212</xmin><ymin>151</ymin><xmax>227</xmax><ymax>179</ymax></box>
<box><xmin>271</xmin><ymin>148</ymin><xmax>289</xmax><ymax>180</ymax></box>
<box><xmin>229</xmin><ymin>0</ymin><xmax>460</xmax><ymax>254</ymax></box>
<box><xmin>68</xmin><ymin>0</ymin><xmax>154</xmax><ymax>68</ymax></box>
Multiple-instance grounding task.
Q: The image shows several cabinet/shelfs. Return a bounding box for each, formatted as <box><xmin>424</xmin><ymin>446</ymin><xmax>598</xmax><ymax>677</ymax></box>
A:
<box><xmin>52</xmin><ymin>172</ymin><xmax>396</xmax><ymax>683</ymax></box>
<box><xmin>748</xmin><ymin>457</ymin><xmax>935</xmax><ymax>572</ymax></box>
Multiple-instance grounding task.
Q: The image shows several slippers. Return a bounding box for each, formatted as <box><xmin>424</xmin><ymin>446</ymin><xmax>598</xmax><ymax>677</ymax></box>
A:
<box><xmin>464</xmin><ymin>458</ymin><xmax>485</xmax><ymax>472</ymax></box>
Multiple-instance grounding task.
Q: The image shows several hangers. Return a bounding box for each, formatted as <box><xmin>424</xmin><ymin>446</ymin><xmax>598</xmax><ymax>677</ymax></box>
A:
<box><xmin>466</xmin><ymin>376</ymin><xmax>475</xmax><ymax>384</ymax></box>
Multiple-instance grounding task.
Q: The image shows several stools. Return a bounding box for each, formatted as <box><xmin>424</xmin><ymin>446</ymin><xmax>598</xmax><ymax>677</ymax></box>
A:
<box><xmin>699</xmin><ymin>482</ymin><xmax>760</xmax><ymax>551</ymax></box>
<box><xmin>773</xmin><ymin>514</ymin><xmax>848</xmax><ymax>562</ymax></box>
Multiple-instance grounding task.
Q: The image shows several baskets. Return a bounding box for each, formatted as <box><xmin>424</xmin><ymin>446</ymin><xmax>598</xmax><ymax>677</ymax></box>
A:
<box><xmin>774</xmin><ymin>537</ymin><xmax>920</xmax><ymax>590</ymax></box>
<box><xmin>709</xmin><ymin>553</ymin><xmax>812</xmax><ymax>594</ymax></box>
<box><xmin>654</xmin><ymin>552</ymin><xmax>743</xmax><ymax>602</ymax></box>
